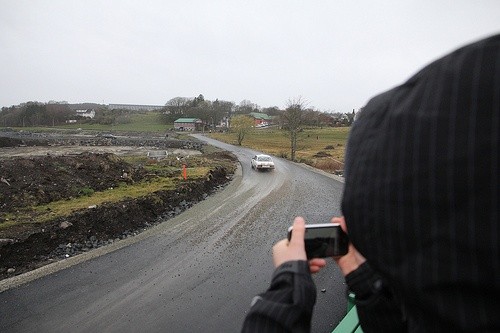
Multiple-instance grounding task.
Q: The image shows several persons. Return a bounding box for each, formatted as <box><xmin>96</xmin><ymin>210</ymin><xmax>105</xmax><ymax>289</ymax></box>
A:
<box><xmin>238</xmin><ymin>32</ymin><xmax>500</xmax><ymax>333</ymax></box>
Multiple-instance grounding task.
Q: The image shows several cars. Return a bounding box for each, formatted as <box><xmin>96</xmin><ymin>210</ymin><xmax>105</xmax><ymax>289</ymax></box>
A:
<box><xmin>251</xmin><ymin>154</ymin><xmax>274</xmax><ymax>171</ymax></box>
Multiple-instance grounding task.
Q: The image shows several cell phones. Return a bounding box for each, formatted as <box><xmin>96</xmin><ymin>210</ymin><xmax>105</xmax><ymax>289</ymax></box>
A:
<box><xmin>287</xmin><ymin>222</ymin><xmax>349</xmax><ymax>261</ymax></box>
